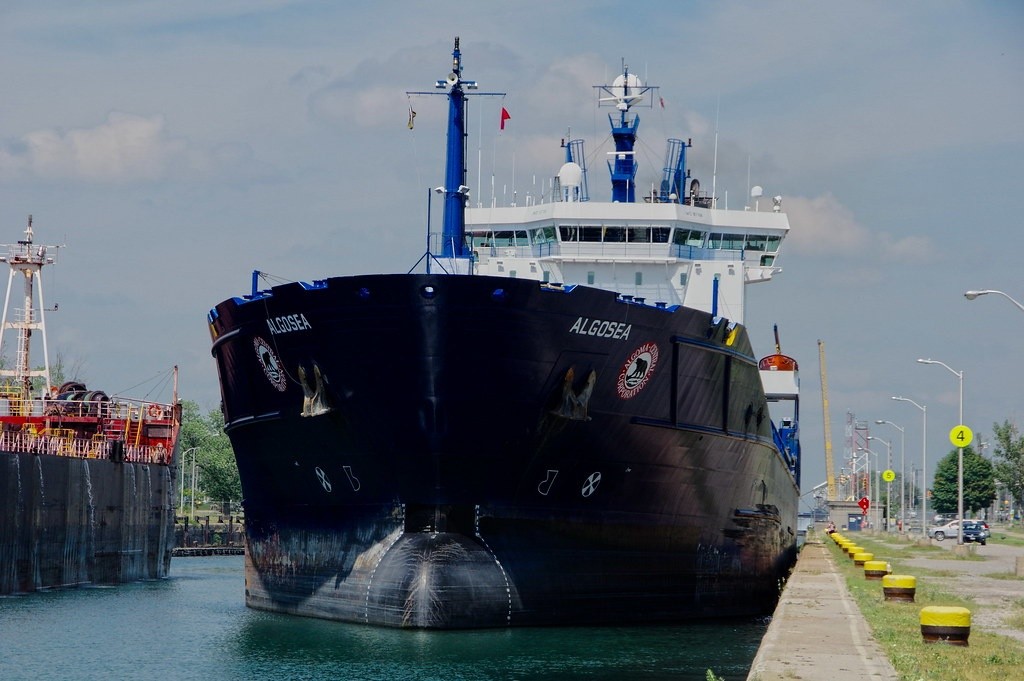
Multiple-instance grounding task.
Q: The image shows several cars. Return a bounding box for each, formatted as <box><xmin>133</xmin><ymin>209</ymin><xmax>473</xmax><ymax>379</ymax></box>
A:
<box><xmin>957</xmin><ymin>521</ymin><xmax>986</xmax><ymax>544</ymax></box>
<box><xmin>928</xmin><ymin>519</ymin><xmax>991</xmax><ymax>541</ymax></box>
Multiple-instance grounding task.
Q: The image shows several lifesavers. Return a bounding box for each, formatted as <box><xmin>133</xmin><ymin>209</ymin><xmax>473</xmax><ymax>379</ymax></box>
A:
<box><xmin>146</xmin><ymin>403</ymin><xmax>162</xmax><ymax>418</ymax></box>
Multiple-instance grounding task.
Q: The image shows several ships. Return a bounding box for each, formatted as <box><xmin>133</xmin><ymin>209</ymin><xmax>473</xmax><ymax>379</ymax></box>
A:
<box><xmin>208</xmin><ymin>28</ymin><xmax>802</xmax><ymax>639</ymax></box>
<box><xmin>2</xmin><ymin>215</ymin><xmax>178</xmax><ymax>611</ymax></box>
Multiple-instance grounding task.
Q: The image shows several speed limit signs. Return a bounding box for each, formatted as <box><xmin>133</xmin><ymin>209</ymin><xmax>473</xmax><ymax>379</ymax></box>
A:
<box><xmin>882</xmin><ymin>470</ymin><xmax>895</xmax><ymax>482</ymax></box>
<box><xmin>949</xmin><ymin>425</ymin><xmax>973</xmax><ymax>448</ymax></box>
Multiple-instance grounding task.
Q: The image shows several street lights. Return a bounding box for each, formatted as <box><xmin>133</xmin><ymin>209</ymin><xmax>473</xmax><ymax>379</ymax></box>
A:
<box><xmin>875</xmin><ymin>418</ymin><xmax>905</xmax><ymax>535</ymax></box>
<box><xmin>917</xmin><ymin>356</ymin><xmax>966</xmax><ymax>546</ymax></box>
<box><xmin>963</xmin><ymin>289</ymin><xmax>1023</xmax><ymax>313</ymax></box>
<box><xmin>891</xmin><ymin>393</ymin><xmax>922</xmax><ymax>539</ymax></box>
<box><xmin>866</xmin><ymin>435</ymin><xmax>890</xmax><ymax>534</ymax></box>
<box><xmin>857</xmin><ymin>447</ymin><xmax>880</xmax><ymax>536</ymax></box>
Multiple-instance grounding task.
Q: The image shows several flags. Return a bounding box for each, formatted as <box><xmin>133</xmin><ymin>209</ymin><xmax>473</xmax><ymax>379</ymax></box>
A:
<box><xmin>408</xmin><ymin>110</ymin><xmax>417</xmax><ymax>129</ymax></box>
<box><xmin>501</xmin><ymin>107</ymin><xmax>511</xmax><ymax>129</ymax></box>
